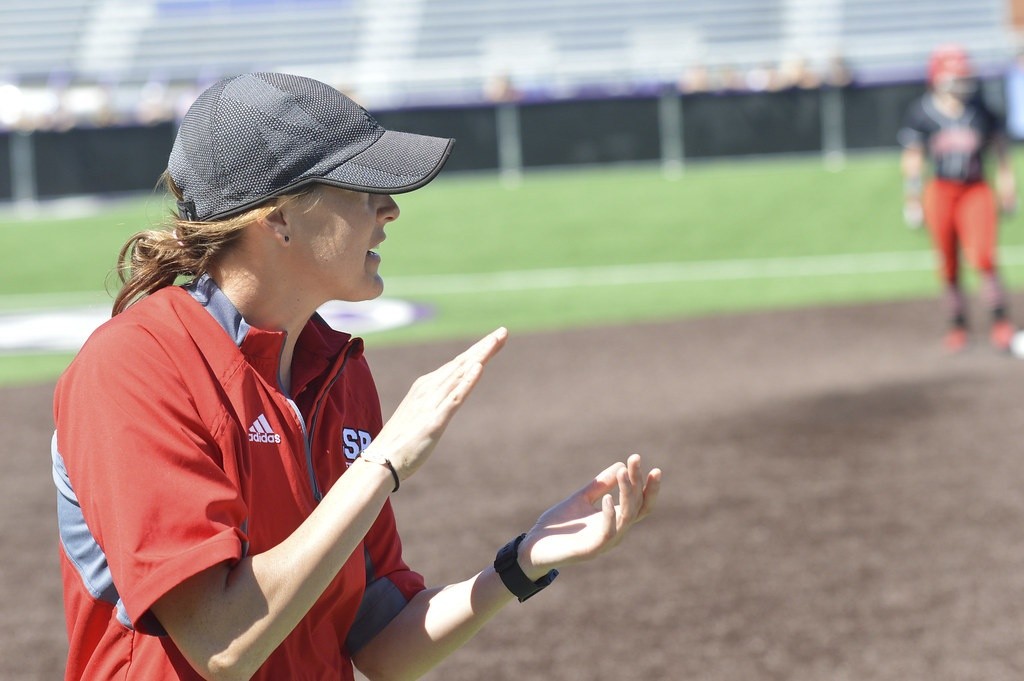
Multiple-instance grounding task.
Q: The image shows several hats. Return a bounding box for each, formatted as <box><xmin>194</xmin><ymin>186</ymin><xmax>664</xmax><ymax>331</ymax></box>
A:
<box><xmin>170</xmin><ymin>72</ymin><xmax>455</xmax><ymax>223</ymax></box>
<box><xmin>929</xmin><ymin>47</ymin><xmax>970</xmax><ymax>77</ymax></box>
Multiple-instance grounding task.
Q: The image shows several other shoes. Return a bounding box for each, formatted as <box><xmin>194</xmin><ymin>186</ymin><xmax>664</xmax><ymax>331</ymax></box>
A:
<box><xmin>988</xmin><ymin>322</ymin><xmax>1013</xmax><ymax>351</ymax></box>
<box><xmin>944</xmin><ymin>330</ymin><xmax>970</xmax><ymax>350</ymax></box>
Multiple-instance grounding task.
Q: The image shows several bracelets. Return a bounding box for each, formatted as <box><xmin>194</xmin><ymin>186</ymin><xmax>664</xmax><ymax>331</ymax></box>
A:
<box><xmin>359</xmin><ymin>452</ymin><xmax>400</xmax><ymax>493</ymax></box>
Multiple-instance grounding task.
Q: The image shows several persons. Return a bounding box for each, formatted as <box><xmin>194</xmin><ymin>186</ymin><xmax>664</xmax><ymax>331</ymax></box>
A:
<box><xmin>899</xmin><ymin>49</ymin><xmax>1024</xmax><ymax>361</ymax></box>
<box><xmin>51</xmin><ymin>72</ymin><xmax>662</xmax><ymax>680</ymax></box>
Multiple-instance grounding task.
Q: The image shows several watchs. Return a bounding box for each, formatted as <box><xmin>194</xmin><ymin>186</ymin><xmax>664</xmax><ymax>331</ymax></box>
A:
<box><xmin>494</xmin><ymin>533</ymin><xmax>559</xmax><ymax>604</ymax></box>
